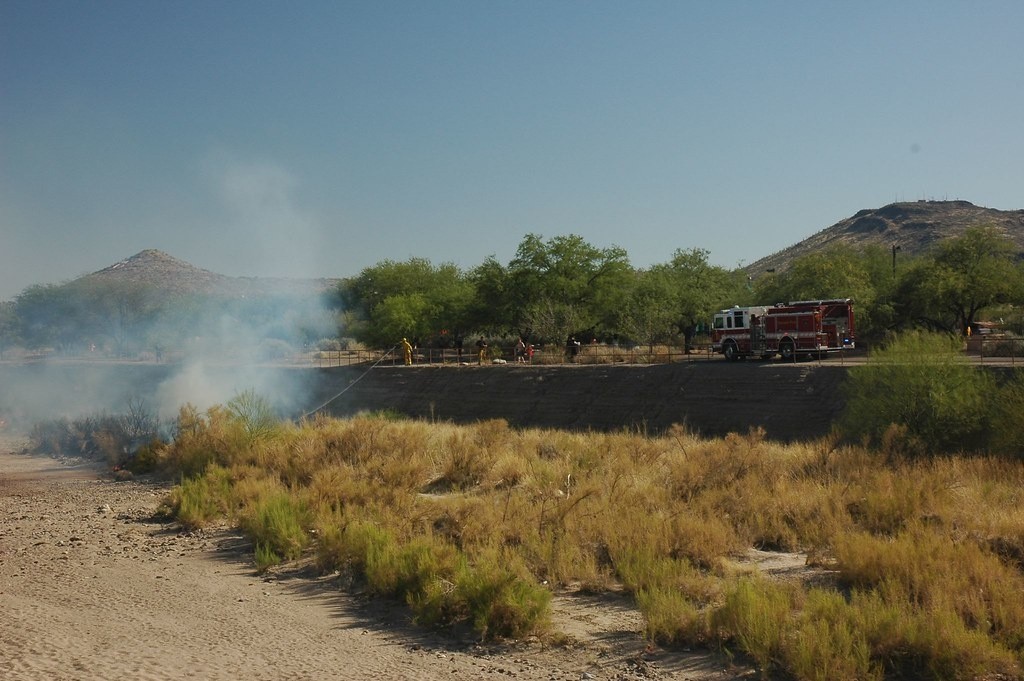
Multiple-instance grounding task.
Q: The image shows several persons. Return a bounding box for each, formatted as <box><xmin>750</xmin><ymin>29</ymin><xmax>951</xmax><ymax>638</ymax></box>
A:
<box><xmin>517</xmin><ymin>339</ymin><xmax>525</xmax><ymax>364</ymax></box>
<box><xmin>476</xmin><ymin>337</ymin><xmax>486</xmax><ymax>365</ymax></box>
<box><xmin>398</xmin><ymin>338</ymin><xmax>412</xmax><ymax>366</ymax></box>
<box><xmin>155</xmin><ymin>344</ymin><xmax>163</xmax><ymax>363</ymax></box>
<box><xmin>455</xmin><ymin>336</ymin><xmax>462</xmax><ymax>359</ymax></box>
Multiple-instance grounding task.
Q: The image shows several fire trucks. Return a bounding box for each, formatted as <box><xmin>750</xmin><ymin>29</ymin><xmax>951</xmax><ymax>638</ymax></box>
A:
<box><xmin>711</xmin><ymin>298</ymin><xmax>856</xmax><ymax>362</ymax></box>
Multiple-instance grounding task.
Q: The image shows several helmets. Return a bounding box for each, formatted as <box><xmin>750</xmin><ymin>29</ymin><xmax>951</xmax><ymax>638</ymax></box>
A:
<box><xmin>402</xmin><ymin>338</ymin><xmax>406</xmax><ymax>341</ymax></box>
